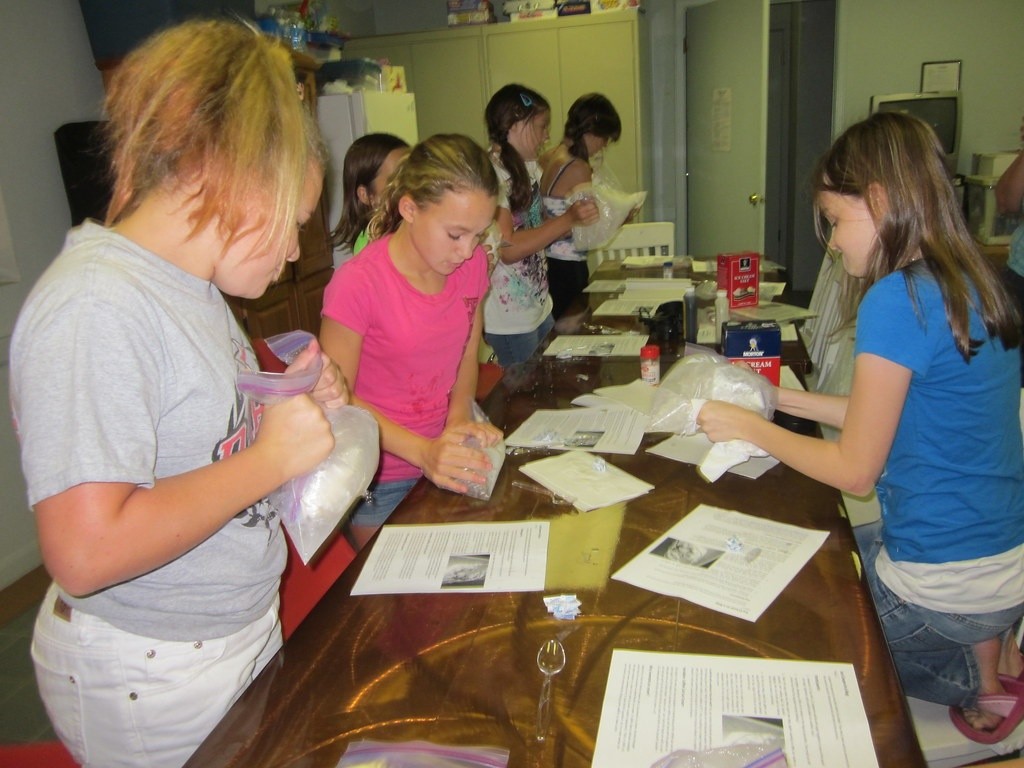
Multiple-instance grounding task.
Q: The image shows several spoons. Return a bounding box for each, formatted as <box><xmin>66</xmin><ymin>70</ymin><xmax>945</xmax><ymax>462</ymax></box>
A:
<box><xmin>536</xmin><ymin>639</ymin><xmax>566</xmax><ymax>740</ymax></box>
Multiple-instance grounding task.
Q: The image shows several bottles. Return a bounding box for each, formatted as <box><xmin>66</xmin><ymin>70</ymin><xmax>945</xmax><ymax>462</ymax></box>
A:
<box><xmin>714</xmin><ymin>289</ymin><xmax>729</xmax><ymax>344</ymax></box>
<box><xmin>663</xmin><ymin>262</ymin><xmax>673</xmax><ymax>278</ymax></box>
<box><xmin>267</xmin><ymin>5</ymin><xmax>304</xmax><ymax>47</ymax></box>
<box><xmin>684</xmin><ymin>287</ymin><xmax>696</xmax><ymax>343</ymax></box>
<box><xmin>641</xmin><ymin>345</ymin><xmax>659</xmax><ymax>384</ymax></box>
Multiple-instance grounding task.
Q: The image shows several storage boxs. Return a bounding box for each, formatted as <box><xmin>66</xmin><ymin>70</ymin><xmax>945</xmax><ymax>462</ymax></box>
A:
<box><xmin>721</xmin><ymin>319</ymin><xmax>780</xmax><ymax>386</ymax></box>
<box><xmin>965</xmin><ymin>176</ymin><xmax>1011</xmax><ymax>245</ymax></box>
<box><xmin>717</xmin><ymin>251</ymin><xmax>759</xmax><ymax>308</ymax></box>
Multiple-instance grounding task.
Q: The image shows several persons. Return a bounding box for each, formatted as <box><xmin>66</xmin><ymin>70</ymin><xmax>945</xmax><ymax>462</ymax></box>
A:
<box><xmin>319</xmin><ymin>133</ymin><xmax>505</xmax><ymax>555</ymax></box>
<box><xmin>697</xmin><ymin>113</ymin><xmax>1024</xmax><ymax>745</ymax></box>
<box><xmin>541</xmin><ymin>93</ymin><xmax>625</xmax><ymax>315</ymax></box>
<box><xmin>995</xmin><ymin>112</ymin><xmax>1024</xmax><ymax>437</ymax></box>
<box><xmin>484</xmin><ymin>84</ymin><xmax>601</xmax><ymax>364</ymax></box>
<box><xmin>10</xmin><ymin>16</ymin><xmax>352</xmax><ymax>768</ymax></box>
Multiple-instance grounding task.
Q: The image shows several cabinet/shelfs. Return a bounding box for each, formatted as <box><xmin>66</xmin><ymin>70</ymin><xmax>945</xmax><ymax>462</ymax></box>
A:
<box><xmin>96</xmin><ymin>36</ymin><xmax>335</xmax><ymax>341</ymax></box>
<box><xmin>343</xmin><ymin>5</ymin><xmax>654</xmax><ymax>276</ymax></box>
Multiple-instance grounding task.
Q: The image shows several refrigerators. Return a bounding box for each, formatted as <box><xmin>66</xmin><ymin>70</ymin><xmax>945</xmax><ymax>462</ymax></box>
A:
<box><xmin>316</xmin><ymin>92</ymin><xmax>419</xmax><ymax>269</ymax></box>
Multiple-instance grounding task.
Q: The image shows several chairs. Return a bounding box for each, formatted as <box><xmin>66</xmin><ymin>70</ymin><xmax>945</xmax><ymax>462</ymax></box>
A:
<box><xmin>907</xmin><ymin>614</ymin><xmax>1024</xmax><ymax>768</ymax></box>
<box><xmin>798</xmin><ymin>226</ymin><xmax>880</xmax><ymax>527</ymax></box>
<box><xmin>597</xmin><ymin>222</ymin><xmax>675</xmax><ymax>266</ymax></box>
<box><xmin>247</xmin><ymin>340</ymin><xmax>356</xmax><ymax>642</ymax></box>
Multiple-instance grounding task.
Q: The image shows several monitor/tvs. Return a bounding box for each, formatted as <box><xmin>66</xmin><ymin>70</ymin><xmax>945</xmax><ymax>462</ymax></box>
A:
<box><xmin>870</xmin><ymin>89</ymin><xmax>963</xmax><ymax>178</ymax></box>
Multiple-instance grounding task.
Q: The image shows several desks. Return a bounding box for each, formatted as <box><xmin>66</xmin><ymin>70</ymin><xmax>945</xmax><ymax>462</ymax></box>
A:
<box><xmin>188</xmin><ymin>257</ymin><xmax>930</xmax><ymax>768</ymax></box>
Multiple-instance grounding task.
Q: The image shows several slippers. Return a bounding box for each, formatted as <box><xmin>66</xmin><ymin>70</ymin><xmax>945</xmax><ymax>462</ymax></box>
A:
<box><xmin>948</xmin><ymin>647</ymin><xmax>1024</xmax><ymax>744</ymax></box>
